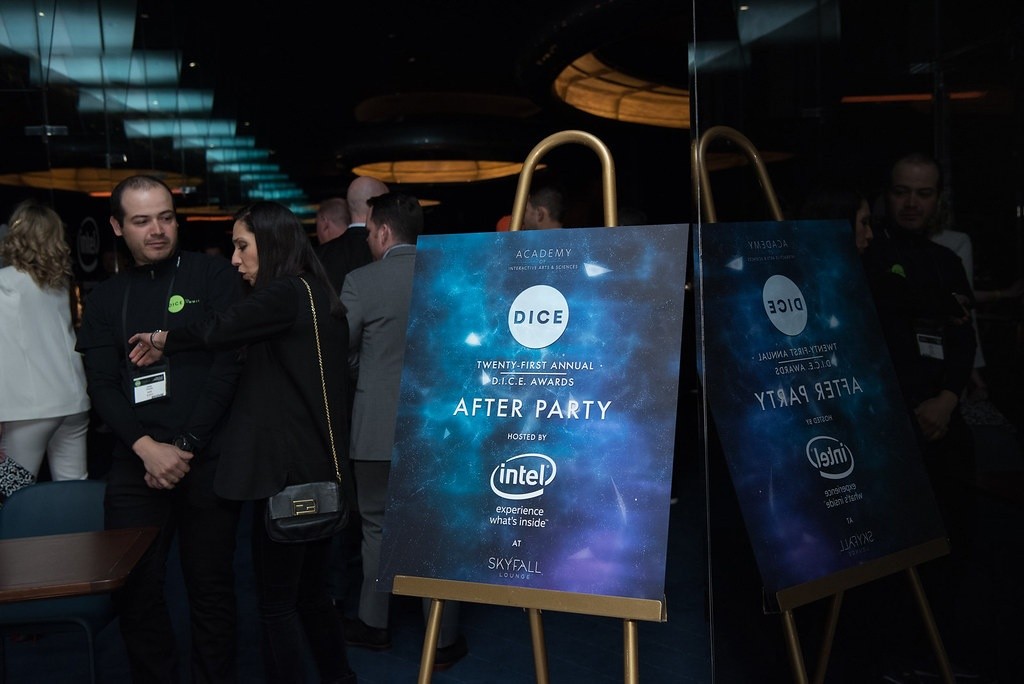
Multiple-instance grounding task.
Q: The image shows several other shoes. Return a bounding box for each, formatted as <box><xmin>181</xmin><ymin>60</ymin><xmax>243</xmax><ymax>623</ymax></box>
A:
<box><xmin>344</xmin><ymin>621</ymin><xmax>392</xmax><ymax>650</ymax></box>
<box><xmin>913</xmin><ymin>655</ymin><xmax>980</xmax><ymax>678</ymax></box>
<box><xmin>418</xmin><ymin>633</ymin><xmax>469</xmax><ymax>672</ymax></box>
<box><xmin>881</xmin><ymin>670</ymin><xmax>936</xmax><ymax>684</ymax></box>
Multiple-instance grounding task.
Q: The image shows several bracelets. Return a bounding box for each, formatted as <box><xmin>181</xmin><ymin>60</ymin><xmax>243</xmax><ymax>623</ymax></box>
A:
<box><xmin>150</xmin><ymin>330</ymin><xmax>163</xmax><ymax>351</ymax></box>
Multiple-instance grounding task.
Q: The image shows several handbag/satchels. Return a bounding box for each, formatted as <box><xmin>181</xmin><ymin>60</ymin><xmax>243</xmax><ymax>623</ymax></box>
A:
<box><xmin>264</xmin><ymin>480</ymin><xmax>349</xmax><ymax>543</ymax></box>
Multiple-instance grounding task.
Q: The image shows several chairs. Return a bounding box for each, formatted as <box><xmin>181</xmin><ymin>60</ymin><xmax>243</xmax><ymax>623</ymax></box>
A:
<box><xmin>0</xmin><ymin>480</ymin><xmax>119</xmax><ymax>684</ymax></box>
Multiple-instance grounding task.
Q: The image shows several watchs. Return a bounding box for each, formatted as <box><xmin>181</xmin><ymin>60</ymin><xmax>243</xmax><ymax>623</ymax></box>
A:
<box><xmin>172</xmin><ymin>436</ymin><xmax>193</xmax><ymax>452</ymax></box>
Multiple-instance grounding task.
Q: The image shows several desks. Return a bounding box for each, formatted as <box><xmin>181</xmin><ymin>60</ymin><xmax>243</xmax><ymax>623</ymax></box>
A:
<box><xmin>0</xmin><ymin>526</ymin><xmax>160</xmax><ymax>604</ymax></box>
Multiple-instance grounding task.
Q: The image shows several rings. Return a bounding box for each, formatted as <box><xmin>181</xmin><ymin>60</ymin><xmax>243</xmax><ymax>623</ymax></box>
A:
<box><xmin>140</xmin><ymin>343</ymin><xmax>145</xmax><ymax>348</ymax></box>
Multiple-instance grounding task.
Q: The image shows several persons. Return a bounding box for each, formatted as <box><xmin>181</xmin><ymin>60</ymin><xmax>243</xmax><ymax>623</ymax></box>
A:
<box><xmin>314</xmin><ymin>177</ymin><xmax>390</xmax><ymax>278</ymax></box>
<box><xmin>339</xmin><ymin>194</ymin><xmax>468</xmax><ymax>670</ymax></box>
<box><xmin>129</xmin><ymin>201</ymin><xmax>354</xmax><ymax>684</ymax></box>
<box><xmin>74</xmin><ymin>175</ymin><xmax>237</xmax><ymax>684</ymax></box>
<box><xmin>799</xmin><ymin>151</ymin><xmax>1024</xmax><ymax>684</ymax></box>
<box><xmin>0</xmin><ymin>201</ymin><xmax>92</xmax><ymax>482</ymax></box>
<box><xmin>316</xmin><ymin>198</ymin><xmax>351</xmax><ymax>244</ymax></box>
<box><xmin>523</xmin><ymin>184</ymin><xmax>572</xmax><ymax>230</ymax></box>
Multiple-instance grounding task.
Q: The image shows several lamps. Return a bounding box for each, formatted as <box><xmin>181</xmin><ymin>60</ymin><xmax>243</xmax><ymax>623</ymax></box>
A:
<box><xmin>0</xmin><ymin>0</ymin><xmax>794</xmax><ymax>223</ymax></box>
